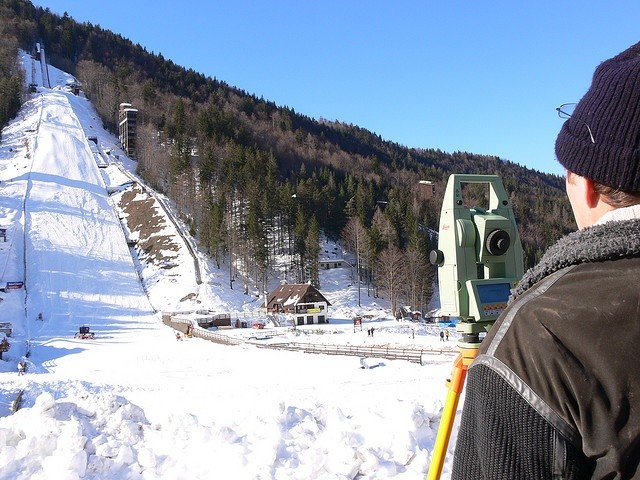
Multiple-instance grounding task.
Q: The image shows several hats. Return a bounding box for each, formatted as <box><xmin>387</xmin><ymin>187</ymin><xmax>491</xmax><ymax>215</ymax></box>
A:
<box><xmin>554</xmin><ymin>39</ymin><xmax>640</xmax><ymax>198</ymax></box>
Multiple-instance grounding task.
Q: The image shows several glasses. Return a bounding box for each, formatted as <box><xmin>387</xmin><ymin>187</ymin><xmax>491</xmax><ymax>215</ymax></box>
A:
<box><xmin>556</xmin><ymin>101</ymin><xmax>598</xmax><ymax>145</ymax></box>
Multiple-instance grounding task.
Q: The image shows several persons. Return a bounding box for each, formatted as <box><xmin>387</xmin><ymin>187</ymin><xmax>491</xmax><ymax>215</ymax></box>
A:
<box><xmin>412</xmin><ymin>329</ymin><xmax>414</xmax><ymax>339</ymax></box>
<box><xmin>446</xmin><ymin>330</ymin><xmax>449</xmax><ymax>340</ymax></box>
<box><xmin>371</xmin><ymin>327</ymin><xmax>374</xmax><ymax>337</ymax></box>
<box><xmin>440</xmin><ymin>331</ymin><xmax>444</xmax><ymax>341</ymax></box>
<box><xmin>453</xmin><ymin>41</ymin><xmax>638</xmax><ymax>477</ymax></box>
